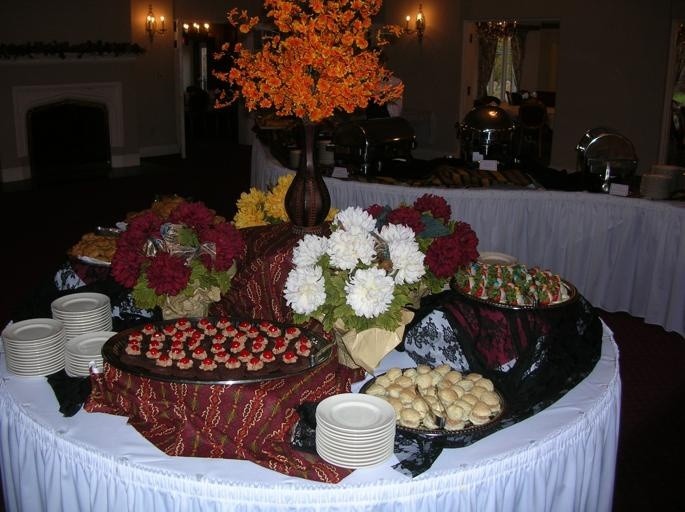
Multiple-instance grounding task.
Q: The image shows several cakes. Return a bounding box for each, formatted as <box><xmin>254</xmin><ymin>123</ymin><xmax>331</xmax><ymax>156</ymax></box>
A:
<box><xmin>366</xmin><ymin>363</ymin><xmax>501</xmax><ymax>431</ymax></box>
<box><xmin>125</xmin><ymin>316</ymin><xmax>312</xmax><ymax>371</ymax></box>
<box><xmin>456</xmin><ymin>258</ymin><xmax>569</xmax><ymax>306</ymax></box>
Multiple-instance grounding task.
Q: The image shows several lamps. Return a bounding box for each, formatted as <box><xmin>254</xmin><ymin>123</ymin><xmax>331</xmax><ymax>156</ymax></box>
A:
<box><xmin>145</xmin><ymin>4</ymin><xmax>165</xmax><ymax>43</ymax></box>
<box><xmin>405</xmin><ymin>4</ymin><xmax>426</xmax><ymax>41</ymax></box>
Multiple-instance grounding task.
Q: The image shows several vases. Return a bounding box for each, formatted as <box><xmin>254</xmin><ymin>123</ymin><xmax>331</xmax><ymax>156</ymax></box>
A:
<box><xmin>285</xmin><ymin>122</ymin><xmax>333</xmax><ymax>223</ymax></box>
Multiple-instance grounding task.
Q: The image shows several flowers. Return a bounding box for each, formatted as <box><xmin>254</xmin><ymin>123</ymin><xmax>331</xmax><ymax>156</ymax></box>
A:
<box><xmin>214</xmin><ymin>0</ymin><xmax>404</xmax><ymax>123</ymax></box>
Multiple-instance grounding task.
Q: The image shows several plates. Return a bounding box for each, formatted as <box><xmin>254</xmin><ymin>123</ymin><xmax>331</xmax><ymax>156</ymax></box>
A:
<box><xmin>314</xmin><ymin>393</ymin><xmax>397</xmax><ymax>471</ymax></box>
<box><xmin>256</xmin><ymin>114</ymin><xmax>292</xmax><ymax>130</ymax></box>
<box><xmin>640</xmin><ymin>164</ymin><xmax>680</xmax><ymax>200</ymax></box>
<box><xmin>476</xmin><ymin>251</ymin><xmax>517</xmax><ymax>268</ymax></box>
<box><xmin>3</xmin><ymin>292</ymin><xmax>117</xmax><ymax>376</ymax></box>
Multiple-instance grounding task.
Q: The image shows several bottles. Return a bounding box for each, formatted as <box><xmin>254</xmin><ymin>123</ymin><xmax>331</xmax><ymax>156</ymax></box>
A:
<box><xmin>281</xmin><ymin>129</ymin><xmax>339</xmax><ymax>173</ymax></box>
